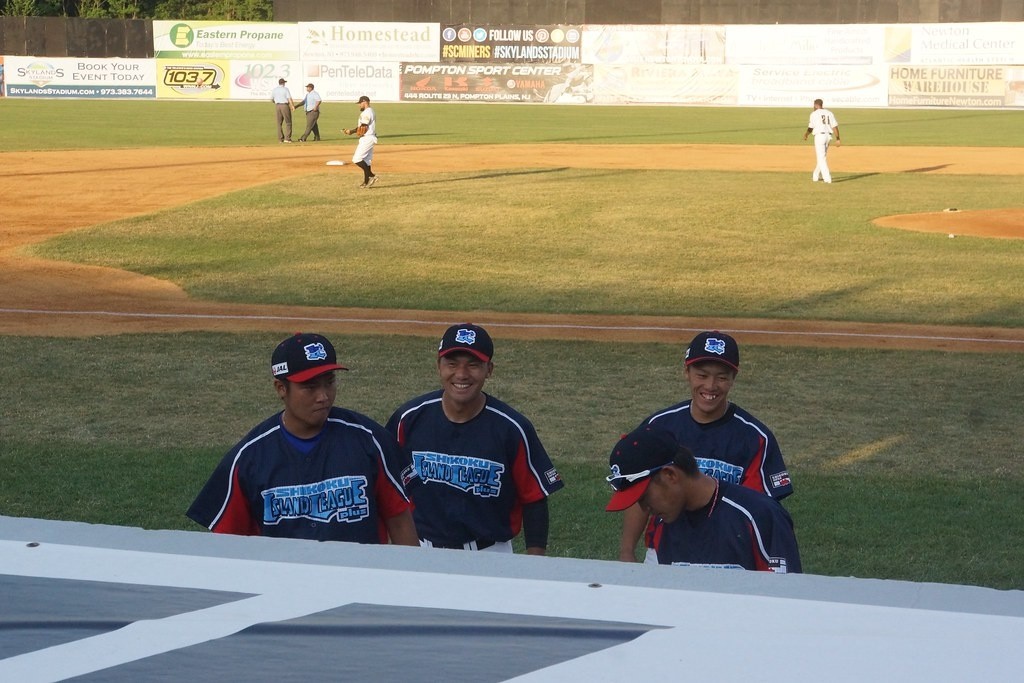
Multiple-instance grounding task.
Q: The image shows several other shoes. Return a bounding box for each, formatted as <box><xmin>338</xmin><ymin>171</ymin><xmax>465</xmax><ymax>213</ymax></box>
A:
<box><xmin>284</xmin><ymin>140</ymin><xmax>291</xmax><ymax>143</ymax></box>
<box><xmin>298</xmin><ymin>139</ymin><xmax>303</xmax><ymax>142</ymax></box>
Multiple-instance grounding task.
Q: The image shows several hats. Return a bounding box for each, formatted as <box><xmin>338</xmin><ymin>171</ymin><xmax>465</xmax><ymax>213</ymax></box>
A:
<box><xmin>356</xmin><ymin>96</ymin><xmax>370</xmax><ymax>104</ymax></box>
<box><xmin>271</xmin><ymin>331</ymin><xmax>349</xmax><ymax>383</ymax></box>
<box><xmin>685</xmin><ymin>329</ymin><xmax>739</xmax><ymax>374</ymax></box>
<box><xmin>438</xmin><ymin>321</ymin><xmax>493</xmax><ymax>363</ymax></box>
<box><xmin>305</xmin><ymin>84</ymin><xmax>314</xmax><ymax>88</ymax></box>
<box><xmin>279</xmin><ymin>78</ymin><xmax>287</xmax><ymax>83</ymax></box>
<box><xmin>605</xmin><ymin>425</ymin><xmax>679</xmax><ymax>512</ymax></box>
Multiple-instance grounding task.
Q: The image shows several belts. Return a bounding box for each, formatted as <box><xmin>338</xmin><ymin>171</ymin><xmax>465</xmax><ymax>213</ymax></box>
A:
<box><xmin>277</xmin><ymin>103</ymin><xmax>288</xmax><ymax>105</ymax></box>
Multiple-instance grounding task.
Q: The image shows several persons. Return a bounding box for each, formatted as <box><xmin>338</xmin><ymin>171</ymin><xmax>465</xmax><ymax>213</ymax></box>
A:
<box><xmin>384</xmin><ymin>321</ymin><xmax>566</xmax><ymax>557</ymax></box>
<box><xmin>294</xmin><ymin>82</ymin><xmax>322</xmax><ymax>142</ymax></box>
<box><xmin>271</xmin><ymin>79</ymin><xmax>295</xmax><ymax>143</ymax></box>
<box><xmin>185</xmin><ymin>332</ymin><xmax>421</xmax><ymax>546</ymax></box>
<box><xmin>803</xmin><ymin>99</ymin><xmax>841</xmax><ymax>183</ymax></box>
<box><xmin>618</xmin><ymin>330</ymin><xmax>797</xmax><ymax>563</ymax></box>
<box><xmin>340</xmin><ymin>95</ymin><xmax>380</xmax><ymax>188</ymax></box>
<box><xmin>605</xmin><ymin>423</ymin><xmax>802</xmax><ymax>574</ymax></box>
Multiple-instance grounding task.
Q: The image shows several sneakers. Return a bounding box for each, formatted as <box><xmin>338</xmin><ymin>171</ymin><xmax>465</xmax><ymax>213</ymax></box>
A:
<box><xmin>360</xmin><ymin>182</ymin><xmax>367</xmax><ymax>189</ymax></box>
<box><xmin>366</xmin><ymin>175</ymin><xmax>379</xmax><ymax>188</ymax></box>
